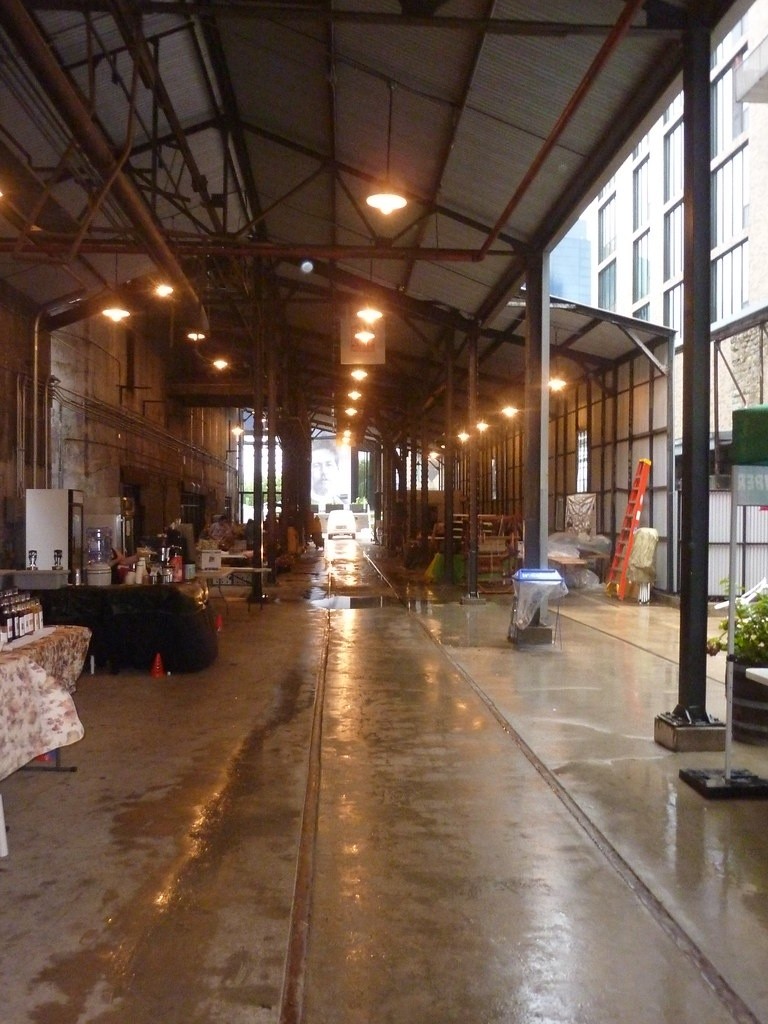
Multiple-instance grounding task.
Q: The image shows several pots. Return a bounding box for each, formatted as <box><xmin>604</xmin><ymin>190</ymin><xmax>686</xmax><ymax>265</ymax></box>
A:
<box><xmin>86</xmin><ymin>562</ymin><xmax>111</xmax><ymax>585</ymax></box>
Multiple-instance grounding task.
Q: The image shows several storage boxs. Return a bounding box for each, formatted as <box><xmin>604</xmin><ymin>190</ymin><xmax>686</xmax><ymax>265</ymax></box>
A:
<box><xmin>10</xmin><ymin>569</ymin><xmax>72</xmax><ymax>590</ymax></box>
<box><xmin>234</xmin><ymin>573</ymin><xmax>251</xmax><ymax>585</ymax></box>
<box><xmin>194</xmin><ymin>548</ymin><xmax>222</xmax><ymax>572</ymax></box>
<box><xmin>213</xmin><ymin>575</ymin><xmax>232</xmax><ymax>585</ymax></box>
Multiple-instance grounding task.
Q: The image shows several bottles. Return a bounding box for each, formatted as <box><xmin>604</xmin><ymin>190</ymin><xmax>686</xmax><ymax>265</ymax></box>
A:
<box><xmin>163</xmin><ymin>556</ymin><xmax>173</xmax><ymax>583</ymax></box>
<box><xmin>183</xmin><ymin>560</ymin><xmax>195</xmax><ymax>581</ymax></box>
<box><xmin>73</xmin><ymin>568</ymin><xmax>81</xmax><ymax>586</ymax></box>
<box><xmin>0</xmin><ymin>586</ymin><xmax>43</xmax><ymax>643</ymax></box>
<box><xmin>170</xmin><ymin>553</ymin><xmax>182</xmax><ymax>582</ymax></box>
<box><xmin>86</xmin><ymin>527</ymin><xmax>111</xmax><ymax>563</ymax></box>
<box><xmin>125</xmin><ymin>572</ymin><xmax>135</xmax><ymax>585</ymax></box>
<box><xmin>135</xmin><ymin>557</ymin><xmax>146</xmax><ymax>584</ymax></box>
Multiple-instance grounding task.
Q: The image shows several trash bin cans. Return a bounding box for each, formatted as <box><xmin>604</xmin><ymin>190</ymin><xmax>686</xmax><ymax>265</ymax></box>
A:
<box><xmin>512</xmin><ymin>567</ymin><xmax>568</xmax><ymax>656</ymax></box>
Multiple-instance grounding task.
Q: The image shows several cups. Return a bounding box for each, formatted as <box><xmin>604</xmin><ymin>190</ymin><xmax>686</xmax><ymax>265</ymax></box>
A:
<box><xmin>149</xmin><ymin>575</ymin><xmax>158</xmax><ymax>585</ymax></box>
<box><xmin>162</xmin><ymin>575</ymin><xmax>170</xmax><ymax>583</ymax></box>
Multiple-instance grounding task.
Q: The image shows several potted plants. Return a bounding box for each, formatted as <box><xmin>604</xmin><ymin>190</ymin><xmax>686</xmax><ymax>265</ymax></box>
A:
<box><xmin>706</xmin><ymin>578</ymin><xmax>768</xmax><ymax>747</ymax></box>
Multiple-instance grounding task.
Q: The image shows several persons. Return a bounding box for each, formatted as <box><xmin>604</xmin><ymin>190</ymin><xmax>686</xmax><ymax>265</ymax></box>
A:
<box><xmin>207</xmin><ymin>516</ymin><xmax>228</xmax><ymax>542</ymax></box>
<box><xmin>310</xmin><ymin>439</ymin><xmax>344</xmax><ymax>508</ymax></box>
<box><xmin>103</xmin><ymin>526</ymin><xmax>123</xmax><ymax>585</ymax></box>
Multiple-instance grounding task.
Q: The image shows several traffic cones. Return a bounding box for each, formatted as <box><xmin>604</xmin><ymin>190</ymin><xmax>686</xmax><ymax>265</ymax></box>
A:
<box><xmin>150</xmin><ymin>655</ymin><xmax>166</xmax><ymax>678</ymax></box>
<box><xmin>212</xmin><ymin>611</ymin><xmax>225</xmax><ymax>631</ymax></box>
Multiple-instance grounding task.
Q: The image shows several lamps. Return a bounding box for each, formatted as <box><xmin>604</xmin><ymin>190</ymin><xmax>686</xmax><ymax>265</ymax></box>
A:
<box><xmin>356</xmin><ymin>257</ymin><xmax>383</xmax><ymax>324</ymax></box>
<box><xmin>364</xmin><ymin>81</ymin><xmax>408</xmax><ymax>215</ymax></box>
<box><xmin>547</xmin><ymin>331</ymin><xmax>567</xmax><ymax>390</ymax></box>
<box><xmin>501</xmin><ymin>363</ymin><xmax>518</xmax><ymax>417</ymax></box>
<box><xmin>101</xmin><ymin>249</ymin><xmax>130</xmax><ymax>321</ymax></box>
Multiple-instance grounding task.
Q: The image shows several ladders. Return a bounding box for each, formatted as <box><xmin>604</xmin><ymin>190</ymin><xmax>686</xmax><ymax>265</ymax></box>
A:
<box><xmin>604</xmin><ymin>458</ymin><xmax>652</xmax><ymax>600</ymax></box>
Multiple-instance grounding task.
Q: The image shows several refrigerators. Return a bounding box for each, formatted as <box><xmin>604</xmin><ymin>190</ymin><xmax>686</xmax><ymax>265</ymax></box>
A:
<box><xmin>85</xmin><ymin>496</ymin><xmax>135</xmax><ymax>559</ymax></box>
<box><xmin>25</xmin><ymin>488</ymin><xmax>84</xmax><ymax>585</ymax></box>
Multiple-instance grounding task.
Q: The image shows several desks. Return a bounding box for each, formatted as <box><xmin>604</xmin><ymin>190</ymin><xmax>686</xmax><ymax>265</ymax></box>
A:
<box><xmin>194</xmin><ymin>567</ymin><xmax>272</xmax><ymax>615</ymax></box>
<box><xmin>221</xmin><ymin>555</ymin><xmax>247</xmax><ymax>566</ymax></box>
<box><xmin>0</xmin><ymin>625</ymin><xmax>93</xmax><ymax>781</ymax></box>
<box><xmin>0</xmin><ymin>577</ymin><xmax>219</xmax><ymax>675</ymax></box>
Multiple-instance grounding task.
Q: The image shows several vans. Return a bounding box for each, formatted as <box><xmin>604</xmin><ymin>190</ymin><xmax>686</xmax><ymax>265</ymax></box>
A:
<box><xmin>326</xmin><ymin>509</ymin><xmax>359</xmax><ymax>539</ymax></box>
